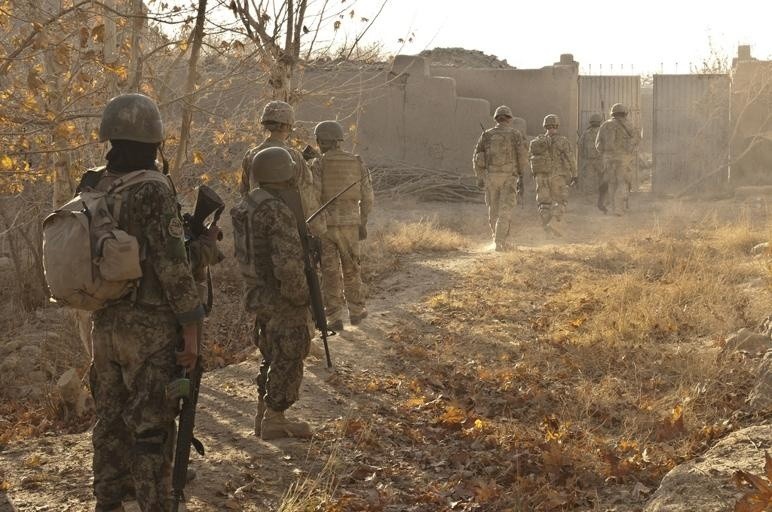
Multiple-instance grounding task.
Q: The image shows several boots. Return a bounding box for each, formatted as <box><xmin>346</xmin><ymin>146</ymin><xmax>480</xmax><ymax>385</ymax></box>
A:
<box><xmin>254</xmin><ymin>399</ymin><xmax>313</xmax><ymax>440</ymax></box>
<box><xmin>547</xmin><ymin>216</ymin><xmax>562</xmax><ymax>237</ymax></box>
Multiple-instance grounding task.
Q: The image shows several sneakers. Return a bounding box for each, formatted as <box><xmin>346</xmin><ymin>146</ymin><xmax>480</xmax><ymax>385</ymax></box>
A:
<box><xmin>349</xmin><ymin>308</ymin><xmax>367</xmax><ymax>325</ymax></box>
<box><xmin>326</xmin><ymin>320</ymin><xmax>343</xmax><ymax>331</ymax></box>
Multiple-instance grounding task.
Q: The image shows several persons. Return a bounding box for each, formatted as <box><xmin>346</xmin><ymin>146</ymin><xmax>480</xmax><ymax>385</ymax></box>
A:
<box><xmin>580</xmin><ymin>113</ymin><xmax>611</xmax><ymax>214</ymax></box>
<box><xmin>530</xmin><ymin>114</ymin><xmax>578</xmax><ymax>231</ymax></box>
<box><xmin>472</xmin><ymin>104</ymin><xmax>529</xmax><ymax>251</ymax></box>
<box><xmin>236</xmin><ymin>147</ymin><xmax>313</xmax><ymax>441</ymax></box>
<box><xmin>74</xmin><ymin>93</ymin><xmax>204</xmax><ymax>512</ymax></box>
<box><xmin>119</xmin><ymin>159</ymin><xmax>221</xmax><ymax>504</ymax></box>
<box><xmin>595</xmin><ymin>102</ymin><xmax>641</xmax><ymax>216</ymax></box>
<box><xmin>239</xmin><ymin>100</ymin><xmax>328</xmax><ymax>338</ymax></box>
<box><xmin>312</xmin><ymin>117</ymin><xmax>376</xmax><ymax>331</ymax></box>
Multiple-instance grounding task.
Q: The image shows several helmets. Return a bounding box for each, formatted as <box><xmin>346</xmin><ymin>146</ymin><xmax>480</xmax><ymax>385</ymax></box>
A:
<box><xmin>494</xmin><ymin>106</ymin><xmax>512</xmax><ymax>118</ymax></box>
<box><xmin>314</xmin><ymin>120</ymin><xmax>344</xmax><ymax>141</ymax></box>
<box><xmin>261</xmin><ymin>101</ymin><xmax>295</xmax><ymax>126</ymax></box>
<box><xmin>252</xmin><ymin>147</ymin><xmax>296</xmax><ymax>182</ymax></box>
<box><xmin>590</xmin><ymin>114</ymin><xmax>603</xmax><ymax>122</ymax></box>
<box><xmin>611</xmin><ymin>103</ymin><xmax>628</xmax><ymax>114</ymax></box>
<box><xmin>100</xmin><ymin>93</ymin><xmax>163</xmax><ymax>143</ymax></box>
<box><xmin>543</xmin><ymin>114</ymin><xmax>560</xmax><ymax>127</ymax></box>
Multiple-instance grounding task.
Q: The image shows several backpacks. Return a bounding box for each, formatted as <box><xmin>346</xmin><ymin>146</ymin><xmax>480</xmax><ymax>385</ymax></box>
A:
<box><xmin>41</xmin><ymin>166</ymin><xmax>172</xmax><ymax>309</ymax></box>
<box><xmin>529</xmin><ymin>134</ymin><xmax>555</xmax><ymax>173</ymax></box>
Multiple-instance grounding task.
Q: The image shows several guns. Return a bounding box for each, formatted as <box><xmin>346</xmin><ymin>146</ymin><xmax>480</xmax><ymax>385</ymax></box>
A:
<box><xmin>164</xmin><ymin>338</ymin><xmax>203</xmax><ymax>512</ymax></box>
<box><xmin>285</xmin><ymin>184</ymin><xmax>333</xmax><ymax>369</ymax></box>
<box><xmin>182</xmin><ymin>184</ymin><xmax>224</xmax><ymax>311</ymax></box>
